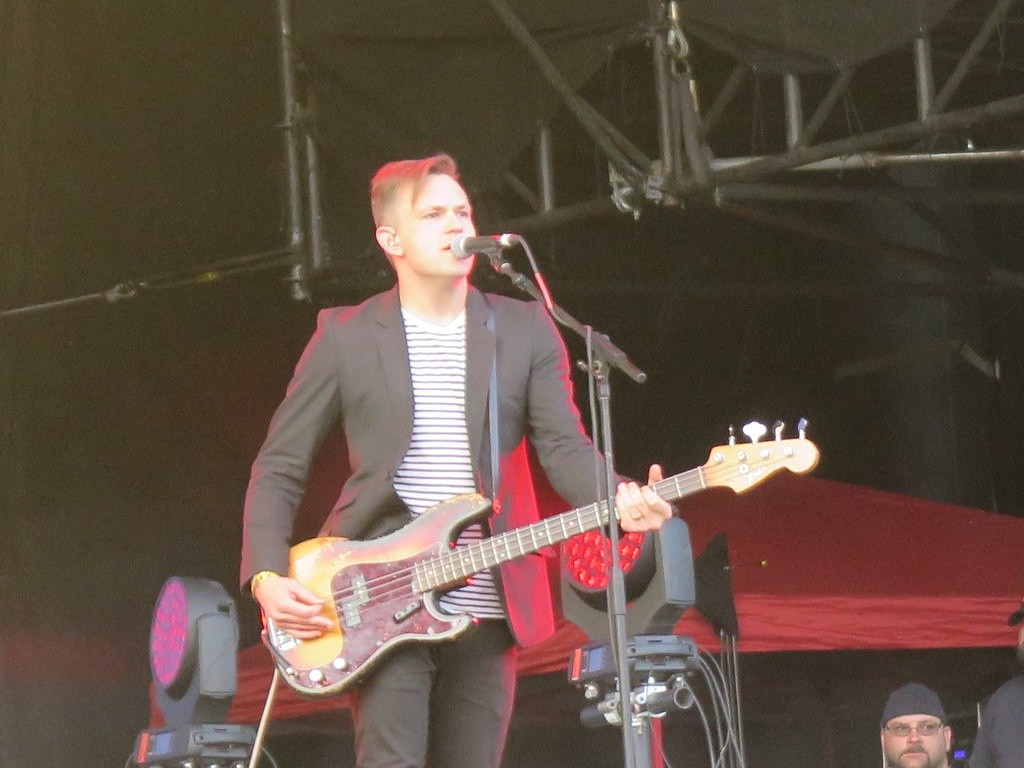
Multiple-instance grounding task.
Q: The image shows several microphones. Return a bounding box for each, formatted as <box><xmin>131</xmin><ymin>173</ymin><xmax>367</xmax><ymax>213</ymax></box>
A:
<box><xmin>449</xmin><ymin>232</ymin><xmax>518</xmax><ymax>262</ymax></box>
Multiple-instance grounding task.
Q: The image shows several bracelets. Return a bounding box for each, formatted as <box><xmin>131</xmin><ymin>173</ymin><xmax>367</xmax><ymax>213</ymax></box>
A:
<box><xmin>251</xmin><ymin>569</ymin><xmax>280</xmax><ymax>598</ymax></box>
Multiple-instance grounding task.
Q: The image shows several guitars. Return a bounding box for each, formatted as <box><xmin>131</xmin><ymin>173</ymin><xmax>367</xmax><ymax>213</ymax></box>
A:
<box><xmin>260</xmin><ymin>417</ymin><xmax>821</xmax><ymax>700</ymax></box>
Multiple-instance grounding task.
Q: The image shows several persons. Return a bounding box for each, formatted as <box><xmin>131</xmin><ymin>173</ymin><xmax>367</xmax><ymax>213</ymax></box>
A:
<box><xmin>237</xmin><ymin>154</ymin><xmax>675</xmax><ymax>768</ymax></box>
<box><xmin>967</xmin><ymin>602</ymin><xmax>1024</xmax><ymax>767</ymax></box>
<box><xmin>882</xmin><ymin>685</ymin><xmax>954</xmax><ymax>768</ymax></box>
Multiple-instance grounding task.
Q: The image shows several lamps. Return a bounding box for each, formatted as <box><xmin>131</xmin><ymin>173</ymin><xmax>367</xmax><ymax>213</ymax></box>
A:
<box><xmin>559</xmin><ymin>510</ymin><xmax>696</xmax><ymax>768</ymax></box>
<box><xmin>124</xmin><ymin>576</ymin><xmax>259</xmax><ymax>768</ymax></box>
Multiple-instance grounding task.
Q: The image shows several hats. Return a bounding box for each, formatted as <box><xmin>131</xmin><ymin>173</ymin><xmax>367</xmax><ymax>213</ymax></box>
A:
<box><xmin>881</xmin><ymin>682</ymin><xmax>948</xmax><ymax>725</ymax></box>
<box><xmin>1007</xmin><ymin>601</ymin><xmax>1024</xmax><ymax>626</ymax></box>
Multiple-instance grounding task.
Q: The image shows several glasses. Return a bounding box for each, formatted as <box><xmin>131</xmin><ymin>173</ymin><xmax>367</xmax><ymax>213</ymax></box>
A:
<box><xmin>884</xmin><ymin>720</ymin><xmax>944</xmax><ymax>735</ymax></box>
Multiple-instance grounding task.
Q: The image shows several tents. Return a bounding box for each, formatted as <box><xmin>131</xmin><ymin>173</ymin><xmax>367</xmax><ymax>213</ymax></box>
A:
<box><xmin>148</xmin><ymin>467</ymin><xmax>1023</xmax><ymax>740</ymax></box>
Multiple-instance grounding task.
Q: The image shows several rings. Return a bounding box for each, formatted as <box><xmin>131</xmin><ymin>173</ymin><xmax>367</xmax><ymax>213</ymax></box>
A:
<box><xmin>632</xmin><ymin>512</ymin><xmax>642</xmax><ymax>522</ymax></box>
